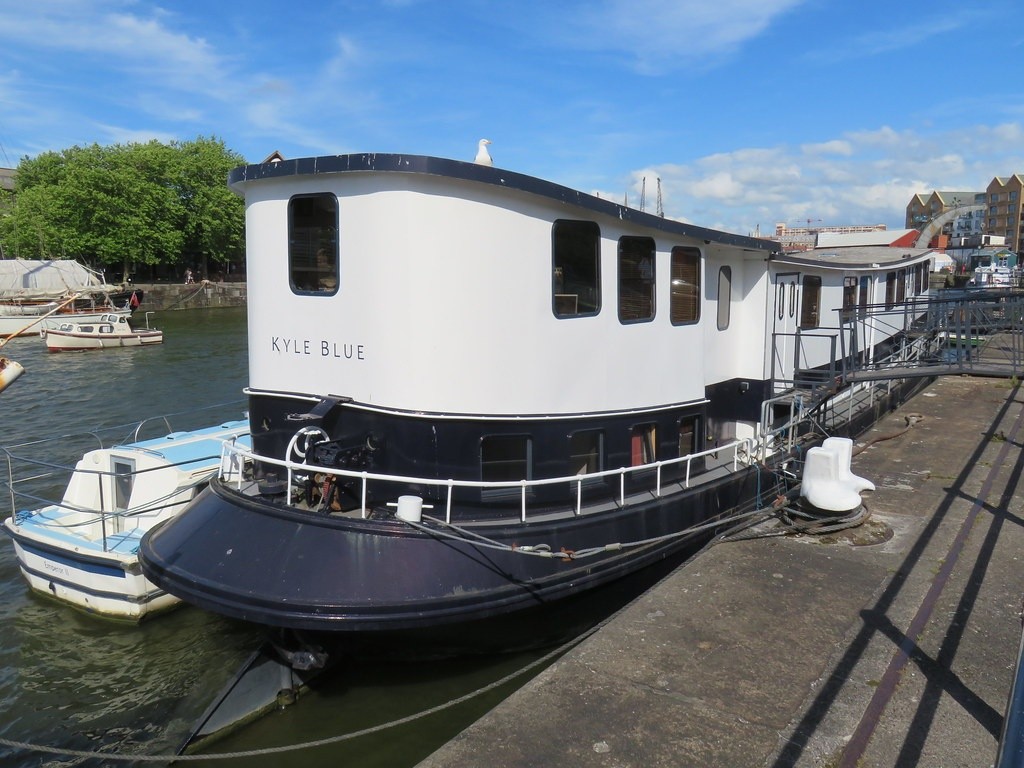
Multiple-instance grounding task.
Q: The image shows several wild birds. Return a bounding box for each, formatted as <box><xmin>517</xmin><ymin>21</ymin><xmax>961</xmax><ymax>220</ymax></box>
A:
<box><xmin>474</xmin><ymin>139</ymin><xmax>494</xmax><ymax>166</ymax></box>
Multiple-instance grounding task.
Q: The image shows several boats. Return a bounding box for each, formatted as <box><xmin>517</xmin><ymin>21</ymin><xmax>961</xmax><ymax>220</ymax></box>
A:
<box><xmin>134</xmin><ymin>153</ymin><xmax>941</xmax><ymax>758</ymax></box>
<box><xmin>38</xmin><ymin>313</ymin><xmax>163</xmax><ymax>352</ymax></box>
<box><xmin>965</xmin><ymin>266</ymin><xmax>1012</xmax><ymax>292</ymax></box>
<box><xmin>0</xmin><ymin>258</ymin><xmax>143</xmax><ymax>314</ymax></box>
<box><xmin>1</xmin><ymin>409</ymin><xmax>251</xmax><ymax>626</ymax></box>
<box><xmin>0</xmin><ymin>298</ymin><xmax>132</xmax><ymax>338</ymax></box>
